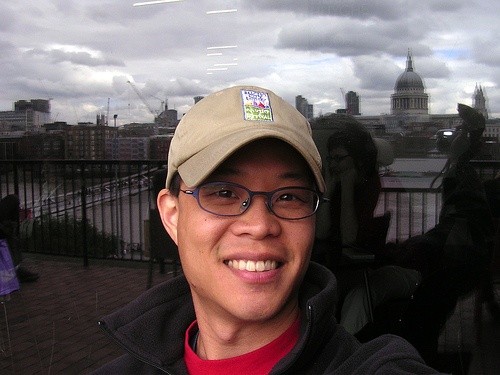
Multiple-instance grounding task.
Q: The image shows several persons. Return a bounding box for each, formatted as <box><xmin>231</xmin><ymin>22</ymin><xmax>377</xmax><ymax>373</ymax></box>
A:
<box><xmin>92</xmin><ymin>85</ymin><xmax>440</xmax><ymax>375</ymax></box>
<box><xmin>309</xmin><ymin>114</ymin><xmax>392</xmax><ymax>273</ymax></box>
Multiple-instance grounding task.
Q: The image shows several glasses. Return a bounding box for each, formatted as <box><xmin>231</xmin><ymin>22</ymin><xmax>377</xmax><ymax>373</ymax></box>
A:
<box><xmin>178</xmin><ymin>188</ymin><xmax>328</xmax><ymax>219</ymax></box>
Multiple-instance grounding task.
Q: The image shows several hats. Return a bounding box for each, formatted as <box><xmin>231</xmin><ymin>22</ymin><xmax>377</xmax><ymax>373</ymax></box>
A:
<box><xmin>166</xmin><ymin>86</ymin><xmax>326</xmax><ymax>195</ymax></box>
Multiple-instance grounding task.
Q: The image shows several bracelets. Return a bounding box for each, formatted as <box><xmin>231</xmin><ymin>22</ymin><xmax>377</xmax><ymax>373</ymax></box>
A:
<box><xmin>322</xmin><ymin>193</ymin><xmax>333</xmax><ymax>201</ymax></box>
